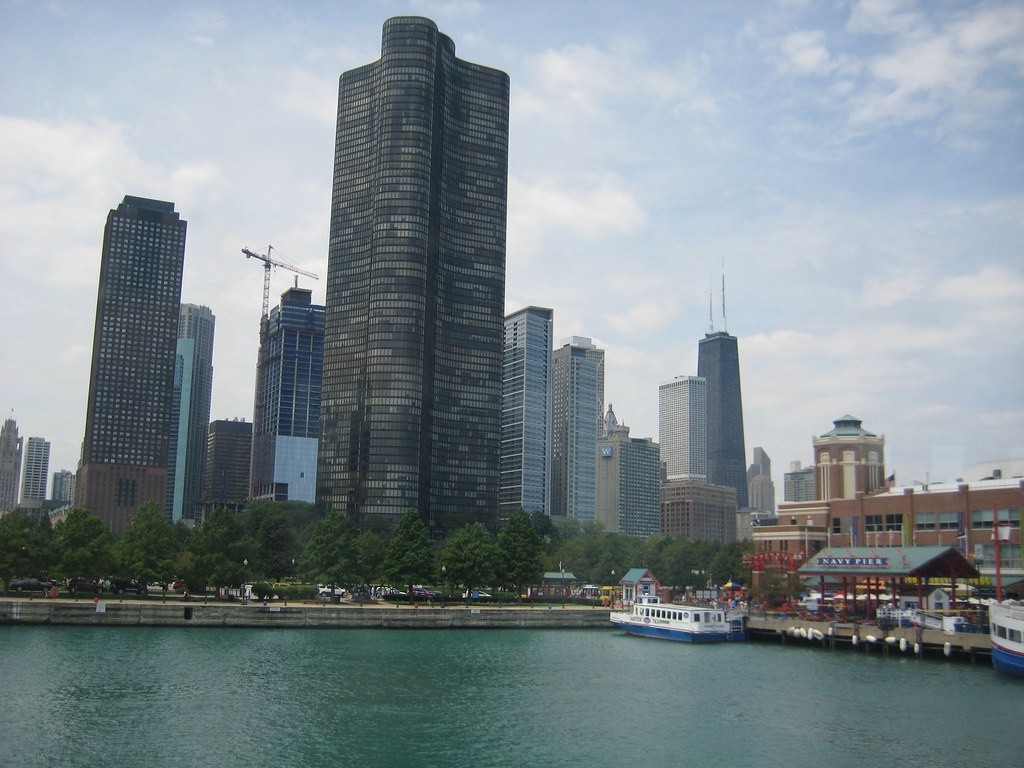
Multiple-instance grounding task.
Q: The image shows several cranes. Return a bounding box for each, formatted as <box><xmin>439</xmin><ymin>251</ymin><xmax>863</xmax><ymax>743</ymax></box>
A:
<box><xmin>241</xmin><ymin>243</ymin><xmax>320</xmax><ymax>501</ymax></box>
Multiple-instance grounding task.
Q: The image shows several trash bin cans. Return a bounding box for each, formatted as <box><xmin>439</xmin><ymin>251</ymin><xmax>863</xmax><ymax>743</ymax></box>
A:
<box><xmin>184</xmin><ymin>606</ymin><xmax>194</xmax><ymax>619</ymax></box>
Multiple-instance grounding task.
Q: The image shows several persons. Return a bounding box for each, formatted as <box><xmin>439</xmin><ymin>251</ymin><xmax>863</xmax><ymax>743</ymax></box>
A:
<box><xmin>682</xmin><ymin>593</ymin><xmax>748</xmax><ymax>612</ymax></box>
<box><xmin>41</xmin><ymin>589</ymin><xmax>49</xmax><ymax>599</ymax></box>
<box><xmin>781</xmin><ymin>602</ymin><xmax>795</xmax><ymax>619</ymax></box>
<box><xmin>371</xmin><ymin>585</ymin><xmax>399</xmax><ymax>599</ymax></box>
<box><xmin>263</xmin><ymin>600</ymin><xmax>267</xmax><ymax>606</ymax></box>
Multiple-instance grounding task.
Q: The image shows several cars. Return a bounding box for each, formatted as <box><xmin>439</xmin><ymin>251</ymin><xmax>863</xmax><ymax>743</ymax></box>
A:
<box><xmin>462</xmin><ymin>589</ymin><xmax>492</xmax><ymax>598</ymax></box>
<box><xmin>172</xmin><ymin>580</ymin><xmax>206</xmax><ymax>592</ymax></box>
<box><xmin>69</xmin><ymin>578</ymin><xmax>106</xmax><ymax>594</ymax></box>
<box><xmin>149</xmin><ymin>581</ymin><xmax>166</xmax><ymax>587</ymax></box>
<box><xmin>110</xmin><ymin>580</ymin><xmax>148</xmax><ymax>595</ymax></box>
<box><xmin>273</xmin><ymin>583</ymin><xmax>290</xmax><ymax>587</ymax></box>
<box><xmin>318</xmin><ymin>584</ymin><xmax>348</xmax><ymax>598</ymax></box>
<box><xmin>406</xmin><ymin>587</ymin><xmax>438</xmax><ymax>603</ymax></box>
<box><xmin>371</xmin><ymin>586</ymin><xmax>406</xmax><ymax>599</ymax></box>
<box><xmin>10</xmin><ymin>578</ymin><xmax>53</xmax><ymax>593</ymax></box>
<box><xmin>571</xmin><ymin>586</ymin><xmax>614</xmax><ymax>604</ymax></box>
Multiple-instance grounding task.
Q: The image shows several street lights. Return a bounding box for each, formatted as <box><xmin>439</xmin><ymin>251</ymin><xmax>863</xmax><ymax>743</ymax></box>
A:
<box><xmin>292</xmin><ymin>559</ymin><xmax>295</xmax><ymax>585</ymax></box>
<box><xmin>561</xmin><ymin>568</ymin><xmax>565</xmax><ymax>604</ymax></box>
<box><xmin>610</xmin><ymin>569</ymin><xmax>615</xmax><ymax>588</ymax></box>
<box><xmin>240</xmin><ymin>558</ymin><xmax>248</xmax><ymax>605</ymax></box>
<box><xmin>441</xmin><ymin>566</ymin><xmax>446</xmax><ymax>607</ymax></box>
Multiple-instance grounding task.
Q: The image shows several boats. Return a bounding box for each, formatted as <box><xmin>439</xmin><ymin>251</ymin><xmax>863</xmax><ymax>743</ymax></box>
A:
<box><xmin>987</xmin><ymin>601</ymin><xmax>1024</xmax><ymax>678</ymax></box>
<box><xmin>609</xmin><ymin>593</ymin><xmax>731</xmax><ymax>643</ymax></box>
